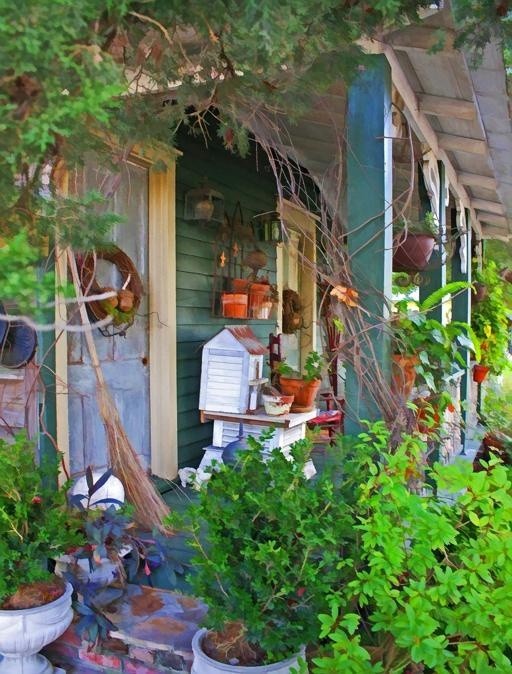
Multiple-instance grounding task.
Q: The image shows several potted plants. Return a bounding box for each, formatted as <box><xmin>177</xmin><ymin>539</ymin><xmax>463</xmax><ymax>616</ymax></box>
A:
<box><xmin>262</xmin><ymin>385</ymin><xmax>291</xmax><ymax>418</ymax></box>
<box><xmin>472</xmin><ymin>313</ymin><xmax>511</xmax><ymax>384</ymax></box>
<box><xmin>0</xmin><ymin>438</ymin><xmax>79</xmax><ymax>674</ymax></box>
<box><xmin>160</xmin><ymin>428</ymin><xmax>312</xmax><ymax>674</ymax></box>
<box><xmin>392</xmin><ymin>209</ymin><xmax>438</xmax><ymax>272</ymax></box>
<box><xmin>283</xmin><ymin>356</ymin><xmax>323</xmax><ymax>412</ymax></box>
